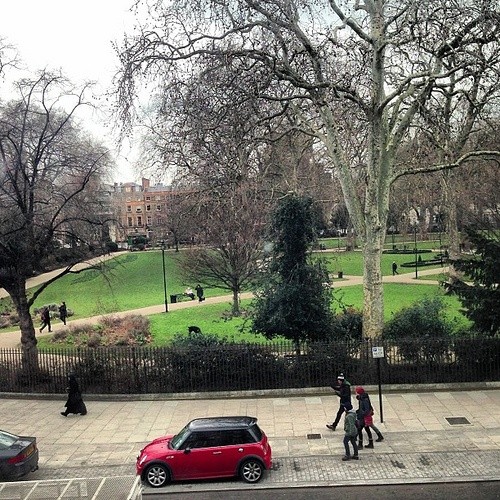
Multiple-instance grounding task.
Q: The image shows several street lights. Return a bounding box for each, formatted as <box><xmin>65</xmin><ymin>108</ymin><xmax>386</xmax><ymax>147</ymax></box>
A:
<box><xmin>160</xmin><ymin>240</ymin><xmax>168</xmax><ymax>312</ymax></box>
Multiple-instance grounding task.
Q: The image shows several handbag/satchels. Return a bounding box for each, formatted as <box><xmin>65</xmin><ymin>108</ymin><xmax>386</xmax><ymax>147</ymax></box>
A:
<box><xmin>364</xmin><ymin>416</ymin><xmax>372</xmax><ymax>426</ymax></box>
<box><xmin>355</xmin><ymin>419</ymin><xmax>360</xmax><ymax>428</ymax></box>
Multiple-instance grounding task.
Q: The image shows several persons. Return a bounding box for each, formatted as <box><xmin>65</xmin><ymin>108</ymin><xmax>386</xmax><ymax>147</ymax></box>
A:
<box><xmin>326</xmin><ymin>373</ymin><xmax>353</xmax><ymax>431</ymax></box>
<box><xmin>393</xmin><ymin>262</ymin><xmax>398</xmax><ymax>275</ymax></box>
<box><xmin>195</xmin><ymin>284</ymin><xmax>203</xmax><ymax>301</ymax></box>
<box><xmin>60</xmin><ymin>375</ymin><xmax>87</xmax><ymax>416</ymax></box>
<box><xmin>342</xmin><ymin>403</ymin><xmax>359</xmax><ymax>461</ymax></box>
<box><xmin>39</xmin><ymin>308</ymin><xmax>52</xmax><ymax>332</ymax></box>
<box><xmin>355</xmin><ymin>387</ymin><xmax>383</xmax><ymax>450</ymax></box>
<box><xmin>187</xmin><ymin>288</ymin><xmax>194</xmax><ymax>300</ymax></box>
<box><xmin>59</xmin><ymin>302</ymin><xmax>66</xmax><ymax>325</ymax></box>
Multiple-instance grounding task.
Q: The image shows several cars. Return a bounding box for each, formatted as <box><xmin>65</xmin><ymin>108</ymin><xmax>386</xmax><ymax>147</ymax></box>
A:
<box><xmin>313</xmin><ymin>229</ymin><xmax>341</xmax><ymax>238</ymax></box>
<box><xmin>1</xmin><ymin>429</ymin><xmax>39</xmax><ymax>482</ymax></box>
<box><xmin>136</xmin><ymin>417</ymin><xmax>272</xmax><ymax>488</ymax></box>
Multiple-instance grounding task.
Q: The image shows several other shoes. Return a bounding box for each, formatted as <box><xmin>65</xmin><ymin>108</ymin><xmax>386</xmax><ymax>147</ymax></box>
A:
<box><xmin>61</xmin><ymin>412</ymin><xmax>68</xmax><ymax>417</ymax></box>
<box><xmin>80</xmin><ymin>411</ymin><xmax>87</xmax><ymax>415</ymax></box>
<box><xmin>342</xmin><ymin>455</ymin><xmax>351</xmax><ymax>461</ymax></box>
<box><xmin>326</xmin><ymin>425</ymin><xmax>335</xmax><ymax>431</ymax></box>
<box><xmin>351</xmin><ymin>455</ymin><xmax>359</xmax><ymax>460</ymax></box>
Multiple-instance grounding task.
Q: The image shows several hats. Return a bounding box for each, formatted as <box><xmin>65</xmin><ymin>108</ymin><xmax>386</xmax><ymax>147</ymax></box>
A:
<box><xmin>356</xmin><ymin>386</ymin><xmax>364</xmax><ymax>394</ymax></box>
<box><xmin>337</xmin><ymin>373</ymin><xmax>345</xmax><ymax>380</ymax></box>
<box><xmin>345</xmin><ymin>404</ymin><xmax>353</xmax><ymax>411</ymax></box>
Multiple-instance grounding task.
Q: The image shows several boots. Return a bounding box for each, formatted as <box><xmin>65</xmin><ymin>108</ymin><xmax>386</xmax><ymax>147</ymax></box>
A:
<box><xmin>357</xmin><ymin>440</ymin><xmax>363</xmax><ymax>450</ymax></box>
<box><xmin>375</xmin><ymin>433</ymin><xmax>383</xmax><ymax>442</ymax></box>
<box><xmin>365</xmin><ymin>440</ymin><xmax>373</xmax><ymax>448</ymax></box>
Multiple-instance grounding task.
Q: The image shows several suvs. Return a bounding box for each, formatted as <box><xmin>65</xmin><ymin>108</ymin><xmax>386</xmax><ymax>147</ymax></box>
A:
<box><xmin>431</xmin><ymin>215</ymin><xmax>448</xmax><ymax>232</ymax></box>
